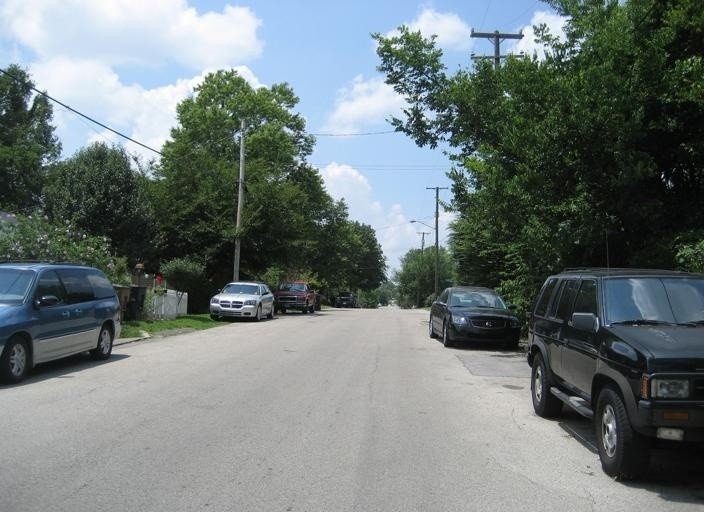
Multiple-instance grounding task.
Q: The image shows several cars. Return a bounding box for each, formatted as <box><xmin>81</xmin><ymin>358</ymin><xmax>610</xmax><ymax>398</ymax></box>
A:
<box><xmin>429</xmin><ymin>286</ymin><xmax>521</xmax><ymax>348</ymax></box>
<box><xmin>210</xmin><ymin>281</ymin><xmax>276</xmax><ymax>322</ymax></box>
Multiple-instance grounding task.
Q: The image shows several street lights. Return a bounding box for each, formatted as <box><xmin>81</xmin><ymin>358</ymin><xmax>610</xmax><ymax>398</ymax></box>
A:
<box><xmin>410</xmin><ymin>221</ymin><xmax>438</xmax><ymax>300</ymax></box>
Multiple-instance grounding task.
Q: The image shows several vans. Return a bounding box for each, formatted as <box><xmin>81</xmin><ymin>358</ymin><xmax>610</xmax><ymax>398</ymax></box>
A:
<box><xmin>0</xmin><ymin>263</ymin><xmax>122</xmax><ymax>383</ymax></box>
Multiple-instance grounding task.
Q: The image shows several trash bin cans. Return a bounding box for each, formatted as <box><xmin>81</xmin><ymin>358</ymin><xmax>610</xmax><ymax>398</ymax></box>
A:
<box><xmin>111</xmin><ymin>284</ymin><xmax>147</xmax><ymax>321</ymax></box>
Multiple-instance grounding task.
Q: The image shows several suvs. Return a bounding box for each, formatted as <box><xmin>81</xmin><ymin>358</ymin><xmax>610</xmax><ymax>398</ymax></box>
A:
<box><xmin>335</xmin><ymin>291</ymin><xmax>356</xmax><ymax>308</ymax></box>
<box><xmin>527</xmin><ymin>268</ymin><xmax>704</xmax><ymax>480</ymax></box>
<box><xmin>274</xmin><ymin>282</ymin><xmax>317</xmax><ymax>314</ymax></box>
